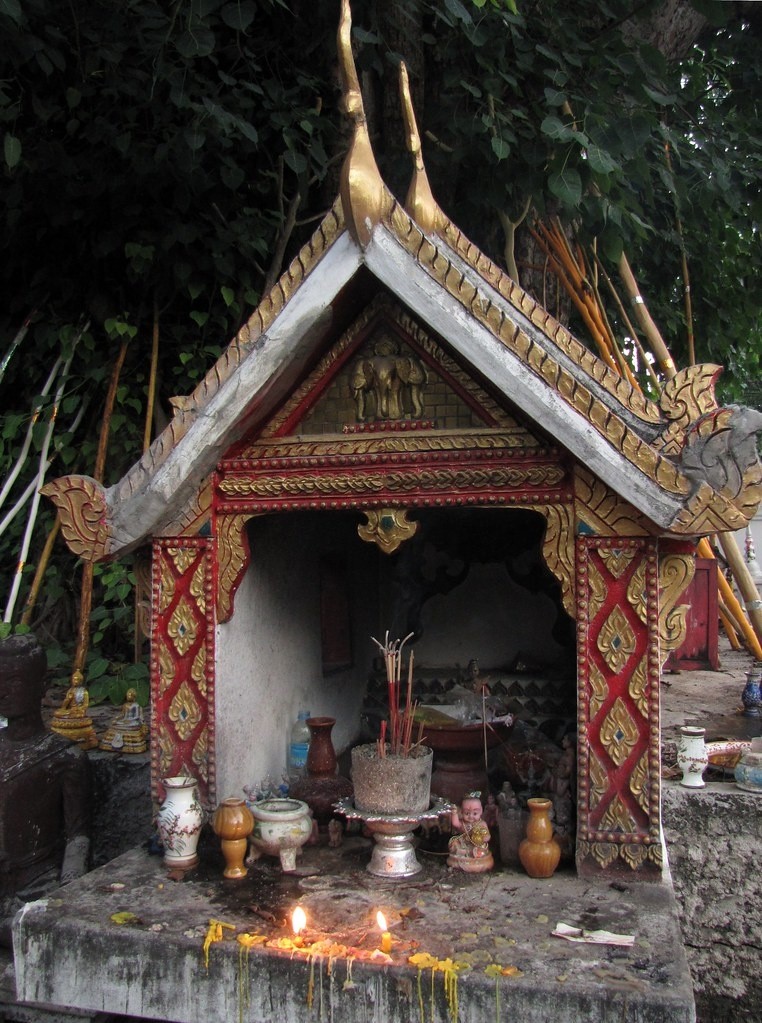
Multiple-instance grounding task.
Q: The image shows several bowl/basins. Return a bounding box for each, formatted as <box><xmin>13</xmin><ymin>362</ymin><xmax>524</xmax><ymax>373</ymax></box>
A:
<box><xmin>734</xmin><ymin>753</ymin><xmax>762</xmax><ymax>794</ymax></box>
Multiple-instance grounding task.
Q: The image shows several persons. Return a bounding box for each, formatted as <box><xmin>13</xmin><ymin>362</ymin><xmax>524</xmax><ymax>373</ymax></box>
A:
<box><xmin>448</xmin><ymin>790</ymin><xmax>492</xmax><ymax>857</ymax></box>
<box><xmin>54</xmin><ymin>669</ymin><xmax>89</xmax><ymax>718</ymax></box>
<box><xmin>112</xmin><ymin>687</ymin><xmax>144</xmax><ymax>731</ymax></box>
<box><xmin>0</xmin><ymin>633</ymin><xmax>94</xmax><ymax>953</ymax></box>
<box><xmin>455</xmin><ymin>657</ymin><xmax>493</xmax><ymax>698</ymax></box>
<box><xmin>422</xmin><ymin>729</ymin><xmax>586</xmax><ymax>838</ymax></box>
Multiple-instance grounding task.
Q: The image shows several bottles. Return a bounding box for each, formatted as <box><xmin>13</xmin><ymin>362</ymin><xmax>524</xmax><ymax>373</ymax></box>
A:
<box><xmin>291</xmin><ymin>717</ymin><xmax>353</xmax><ymax>824</ymax></box>
<box><xmin>741</xmin><ymin>672</ymin><xmax>761</xmax><ymax>718</ymax></box>
<box><xmin>288</xmin><ymin>710</ymin><xmax>310</xmax><ymax>780</ymax></box>
<box><xmin>518</xmin><ymin>798</ymin><xmax>560</xmax><ymax>878</ymax></box>
<box><xmin>213</xmin><ymin>797</ymin><xmax>254</xmax><ymax>879</ymax></box>
<box><xmin>158</xmin><ymin>777</ymin><xmax>204</xmax><ymax>868</ymax></box>
<box><xmin>677</xmin><ymin>726</ymin><xmax>707</xmax><ymax>788</ymax></box>
